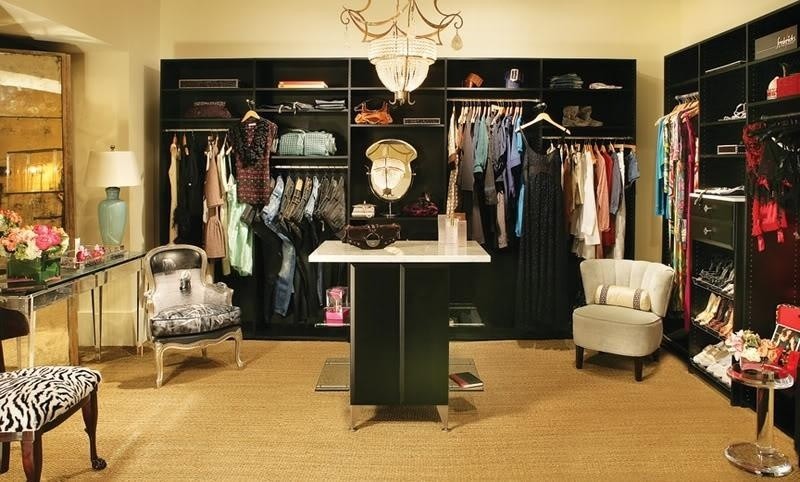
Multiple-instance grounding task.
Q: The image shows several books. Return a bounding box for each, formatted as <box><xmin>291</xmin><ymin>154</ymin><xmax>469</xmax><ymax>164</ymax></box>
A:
<box><xmin>449</xmin><ymin>371</ymin><xmax>484</xmax><ymax>389</ymax></box>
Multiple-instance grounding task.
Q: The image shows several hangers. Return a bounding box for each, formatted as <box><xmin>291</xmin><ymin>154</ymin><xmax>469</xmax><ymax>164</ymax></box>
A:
<box><xmin>654</xmin><ymin>90</ymin><xmax>699</xmax><ymax>127</ymax></box>
<box><xmin>450</xmin><ymin>99</ymin><xmax>636</xmax><ymax>165</ymax></box>
<box><xmin>751</xmin><ymin>111</ymin><xmax>799</xmax><ymax>155</ymax></box>
<box><xmin>240</xmin><ymin>98</ymin><xmax>261</xmax><ymax>123</ymax></box>
<box><xmin>354</xmin><ymin>96</ymin><xmax>396</xmax><ymax>112</ymax></box>
<box><xmin>172</xmin><ymin>129</ymin><xmax>346</xmax><ymax>181</ymax></box>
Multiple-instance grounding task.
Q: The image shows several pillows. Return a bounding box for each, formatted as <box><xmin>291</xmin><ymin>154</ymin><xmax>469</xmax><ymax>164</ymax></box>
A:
<box><xmin>593</xmin><ymin>285</ymin><xmax>651</xmax><ymax>312</ymax></box>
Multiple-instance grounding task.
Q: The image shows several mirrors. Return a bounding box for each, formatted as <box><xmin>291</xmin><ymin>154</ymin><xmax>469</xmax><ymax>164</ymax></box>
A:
<box><xmin>0</xmin><ymin>47</ymin><xmax>79</xmax><ymax>371</ymax></box>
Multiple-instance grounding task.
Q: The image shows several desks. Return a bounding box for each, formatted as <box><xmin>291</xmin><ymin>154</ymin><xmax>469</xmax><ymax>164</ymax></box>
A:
<box><xmin>0</xmin><ymin>250</ymin><xmax>146</xmax><ymax>368</ymax></box>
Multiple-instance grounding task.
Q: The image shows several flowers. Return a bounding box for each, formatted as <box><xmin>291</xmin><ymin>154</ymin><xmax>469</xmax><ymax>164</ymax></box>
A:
<box><xmin>1</xmin><ymin>224</ymin><xmax>69</xmax><ymax>261</ymax></box>
<box><xmin>724</xmin><ymin>327</ymin><xmax>778</xmax><ymax>362</ymax></box>
<box><xmin>0</xmin><ymin>209</ymin><xmax>24</xmax><ymax>236</ymax></box>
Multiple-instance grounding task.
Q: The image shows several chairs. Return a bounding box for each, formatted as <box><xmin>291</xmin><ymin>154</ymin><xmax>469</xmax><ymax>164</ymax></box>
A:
<box><xmin>571</xmin><ymin>258</ymin><xmax>675</xmax><ymax>382</ymax></box>
<box><xmin>142</xmin><ymin>243</ymin><xmax>247</xmax><ymax>389</ymax></box>
<box><xmin>0</xmin><ymin>304</ymin><xmax>107</xmax><ymax>482</ymax></box>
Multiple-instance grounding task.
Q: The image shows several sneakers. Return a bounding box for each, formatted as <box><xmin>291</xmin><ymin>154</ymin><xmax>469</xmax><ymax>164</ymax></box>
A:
<box><xmin>693</xmin><ymin>341</ymin><xmax>732</xmax><ymax>386</ymax></box>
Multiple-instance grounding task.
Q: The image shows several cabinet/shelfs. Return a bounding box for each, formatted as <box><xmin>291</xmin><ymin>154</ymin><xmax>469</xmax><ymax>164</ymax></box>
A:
<box><xmin>307</xmin><ymin>239</ymin><xmax>492</xmax><ymax>431</ymax></box>
<box><xmin>661</xmin><ymin>0</ymin><xmax>800</xmax><ymax>457</ymax></box>
<box><xmin>158</xmin><ymin>57</ymin><xmax>636</xmax><ymax>342</ymax></box>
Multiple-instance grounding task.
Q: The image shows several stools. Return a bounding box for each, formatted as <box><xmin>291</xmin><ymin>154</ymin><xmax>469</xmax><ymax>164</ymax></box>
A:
<box><xmin>724</xmin><ymin>362</ymin><xmax>792</xmax><ymax>477</ymax></box>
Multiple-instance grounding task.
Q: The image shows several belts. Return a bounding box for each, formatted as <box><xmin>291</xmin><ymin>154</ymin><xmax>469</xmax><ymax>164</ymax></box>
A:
<box><xmin>460</xmin><ymin>73</ymin><xmax>486</xmax><ymax>88</ymax></box>
<box><xmin>504</xmin><ymin>68</ymin><xmax>524</xmax><ymax>87</ymax></box>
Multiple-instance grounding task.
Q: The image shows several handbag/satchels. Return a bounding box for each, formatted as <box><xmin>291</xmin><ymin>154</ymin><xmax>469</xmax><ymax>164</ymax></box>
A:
<box><xmin>353</xmin><ymin>96</ymin><xmax>394</xmax><ymax>126</ymax></box>
<box><xmin>401</xmin><ymin>192</ymin><xmax>439</xmax><ymax>216</ymax></box>
<box><xmin>334</xmin><ymin>222</ymin><xmax>400</xmax><ymax>253</ymax></box>
<box><xmin>351</xmin><ymin>201</ymin><xmax>376</xmax><ymax>218</ymax></box>
<box><xmin>275</xmin><ymin>127</ymin><xmax>337</xmax><ymax>157</ymax></box>
<box><xmin>185</xmin><ymin>95</ymin><xmax>232</xmax><ymax>118</ymax></box>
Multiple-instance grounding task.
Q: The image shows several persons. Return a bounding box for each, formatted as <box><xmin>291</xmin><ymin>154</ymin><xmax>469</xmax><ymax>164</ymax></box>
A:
<box><xmin>776</xmin><ymin>328</ymin><xmax>795</xmax><ymax>352</ymax></box>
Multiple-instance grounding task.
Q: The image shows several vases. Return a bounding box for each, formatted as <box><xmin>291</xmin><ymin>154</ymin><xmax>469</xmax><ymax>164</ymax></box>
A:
<box><xmin>739</xmin><ymin>355</ymin><xmax>764</xmax><ymax>372</ymax></box>
<box><xmin>6</xmin><ymin>256</ymin><xmax>60</xmax><ymax>279</ymax></box>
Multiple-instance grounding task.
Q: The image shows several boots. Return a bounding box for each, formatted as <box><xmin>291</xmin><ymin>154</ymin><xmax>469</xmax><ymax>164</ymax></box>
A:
<box><xmin>562</xmin><ymin>105</ymin><xmax>591</xmax><ymax>126</ymax></box>
<box><xmin>579</xmin><ymin>106</ymin><xmax>604</xmax><ymax>127</ymax></box>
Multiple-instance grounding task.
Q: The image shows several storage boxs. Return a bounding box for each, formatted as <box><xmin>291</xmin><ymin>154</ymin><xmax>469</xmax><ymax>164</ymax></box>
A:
<box><xmin>755</xmin><ymin>23</ymin><xmax>799</xmax><ymax>61</ymax></box>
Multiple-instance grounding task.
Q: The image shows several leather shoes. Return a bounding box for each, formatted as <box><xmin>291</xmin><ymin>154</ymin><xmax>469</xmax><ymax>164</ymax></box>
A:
<box><xmin>694</xmin><ymin>257</ymin><xmax>735</xmax><ymax>295</ymax></box>
<box><xmin>693</xmin><ymin>293</ymin><xmax>734</xmax><ymax>337</ymax></box>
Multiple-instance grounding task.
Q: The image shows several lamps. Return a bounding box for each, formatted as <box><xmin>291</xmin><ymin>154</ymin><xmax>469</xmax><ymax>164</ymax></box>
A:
<box><xmin>340</xmin><ymin>0</ymin><xmax>464</xmax><ymax>106</ymax></box>
<box><xmin>82</xmin><ymin>144</ymin><xmax>141</xmax><ymax>250</ymax></box>
